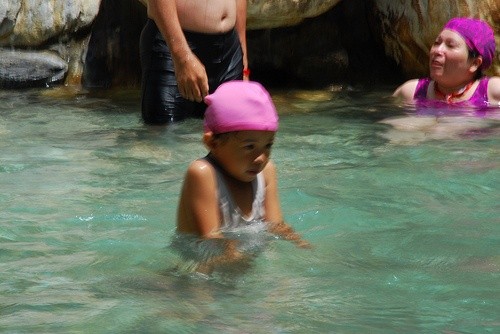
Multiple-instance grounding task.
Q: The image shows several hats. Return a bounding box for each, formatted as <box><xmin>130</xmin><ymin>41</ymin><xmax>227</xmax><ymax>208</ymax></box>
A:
<box><xmin>204</xmin><ymin>81</ymin><xmax>279</xmax><ymax>133</ymax></box>
<box><xmin>445</xmin><ymin>17</ymin><xmax>495</xmax><ymax>72</ymax></box>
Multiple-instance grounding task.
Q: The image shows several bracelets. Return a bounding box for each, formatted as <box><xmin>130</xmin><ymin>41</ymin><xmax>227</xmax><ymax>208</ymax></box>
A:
<box><xmin>243</xmin><ymin>69</ymin><xmax>249</xmax><ymax>77</ymax></box>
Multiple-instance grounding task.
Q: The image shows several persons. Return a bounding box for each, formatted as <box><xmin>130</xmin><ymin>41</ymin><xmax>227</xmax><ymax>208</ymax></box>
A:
<box><xmin>176</xmin><ymin>82</ymin><xmax>311</xmax><ymax>265</ymax></box>
<box><xmin>139</xmin><ymin>0</ymin><xmax>249</xmax><ymax>127</ymax></box>
<box><xmin>380</xmin><ymin>18</ymin><xmax>500</xmax><ymax>130</ymax></box>
